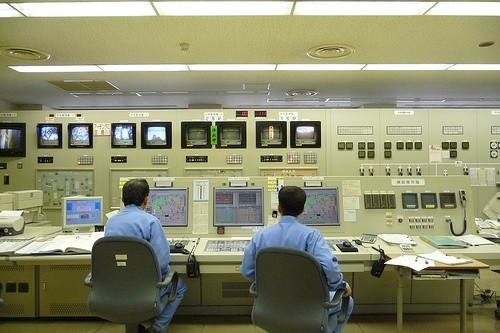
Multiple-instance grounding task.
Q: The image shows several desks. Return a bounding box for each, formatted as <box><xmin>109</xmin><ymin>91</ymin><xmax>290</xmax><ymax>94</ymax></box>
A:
<box><xmin>386</xmin><ymin>253</ymin><xmax>490</xmax><ymax>333</ymax></box>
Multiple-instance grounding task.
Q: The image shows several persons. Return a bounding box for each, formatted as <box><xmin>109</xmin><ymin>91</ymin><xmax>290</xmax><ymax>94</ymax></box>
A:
<box><xmin>240</xmin><ymin>186</ymin><xmax>354</xmax><ymax>333</ymax></box>
<box><xmin>104</xmin><ymin>179</ymin><xmax>188</xmax><ymax>333</ymax></box>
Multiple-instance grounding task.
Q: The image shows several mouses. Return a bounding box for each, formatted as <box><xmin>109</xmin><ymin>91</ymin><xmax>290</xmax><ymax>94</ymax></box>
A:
<box><xmin>343</xmin><ymin>241</ymin><xmax>352</xmax><ymax>247</ymax></box>
<box><xmin>175</xmin><ymin>243</ymin><xmax>182</xmax><ymax>248</ymax></box>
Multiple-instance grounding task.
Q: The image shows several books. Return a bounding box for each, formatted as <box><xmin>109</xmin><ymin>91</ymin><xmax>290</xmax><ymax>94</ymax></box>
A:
<box><xmin>420</xmin><ymin>236</ymin><xmax>465</xmax><ymax>249</ymax></box>
<box><xmin>39</xmin><ymin>240</ymin><xmax>91</xmax><ymax>253</ymax></box>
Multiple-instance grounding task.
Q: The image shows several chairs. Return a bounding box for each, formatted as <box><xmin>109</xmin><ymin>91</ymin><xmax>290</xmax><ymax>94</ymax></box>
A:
<box><xmin>85</xmin><ymin>237</ymin><xmax>179</xmax><ymax>333</ymax></box>
<box><xmin>251</xmin><ymin>247</ymin><xmax>351</xmax><ymax>333</ymax></box>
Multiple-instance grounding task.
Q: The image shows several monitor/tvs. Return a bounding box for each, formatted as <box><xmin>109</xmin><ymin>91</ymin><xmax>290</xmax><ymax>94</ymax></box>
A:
<box><xmin>181</xmin><ymin>121</ymin><xmax>212</xmax><ymax>148</ymax></box>
<box><xmin>212</xmin><ymin>187</ymin><xmax>266</xmax><ymax>227</ymax></box>
<box><xmin>297</xmin><ymin>186</ymin><xmax>340</xmax><ymax>226</ymax></box>
<box><xmin>141</xmin><ymin>121</ymin><xmax>172</xmax><ymax>149</ymax></box>
<box><xmin>256</xmin><ymin>121</ymin><xmax>288</xmax><ymax>148</ymax></box>
<box><xmin>0</xmin><ymin>122</ymin><xmax>27</xmax><ymax>158</ymax></box>
<box><xmin>37</xmin><ymin>122</ymin><xmax>63</xmax><ymax>148</ymax></box>
<box><xmin>62</xmin><ymin>196</ymin><xmax>105</xmax><ymax>227</ymax></box>
<box><xmin>68</xmin><ymin>122</ymin><xmax>93</xmax><ymax>148</ymax></box>
<box><xmin>140</xmin><ymin>186</ymin><xmax>190</xmax><ymax>228</ymax></box>
<box><xmin>214</xmin><ymin>121</ymin><xmax>246</xmax><ymax>148</ymax></box>
<box><xmin>111</xmin><ymin>122</ymin><xmax>136</xmax><ymax>148</ymax></box>
<box><xmin>290</xmin><ymin>121</ymin><xmax>321</xmax><ymax>149</ymax></box>
